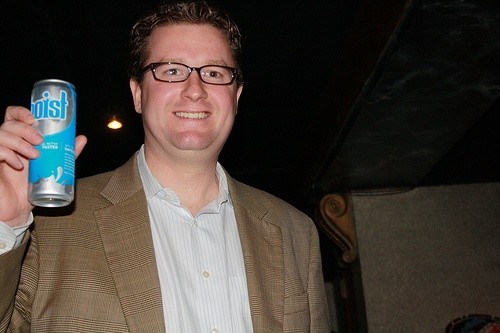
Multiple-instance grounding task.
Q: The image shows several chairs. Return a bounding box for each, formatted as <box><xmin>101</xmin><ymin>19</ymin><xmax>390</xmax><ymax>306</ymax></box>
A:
<box><xmin>315</xmin><ymin>181</ymin><xmax>500</xmax><ymax>333</ymax></box>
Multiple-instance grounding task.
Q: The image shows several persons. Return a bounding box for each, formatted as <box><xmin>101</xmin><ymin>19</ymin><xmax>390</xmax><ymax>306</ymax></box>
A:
<box><xmin>1</xmin><ymin>0</ymin><xmax>332</xmax><ymax>333</ymax></box>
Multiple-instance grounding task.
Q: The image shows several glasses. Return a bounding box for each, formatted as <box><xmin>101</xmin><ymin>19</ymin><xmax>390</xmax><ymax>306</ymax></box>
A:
<box><xmin>140</xmin><ymin>62</ymin><xmax>239</xmax><ymax>85</ymax></box>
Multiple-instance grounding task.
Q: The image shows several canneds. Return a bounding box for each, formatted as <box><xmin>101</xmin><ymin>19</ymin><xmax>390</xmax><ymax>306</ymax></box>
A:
<box><xmin>29</xmin><ymin>78</ymin><xmax>76</xmax><ymax>208</ymax></box>
